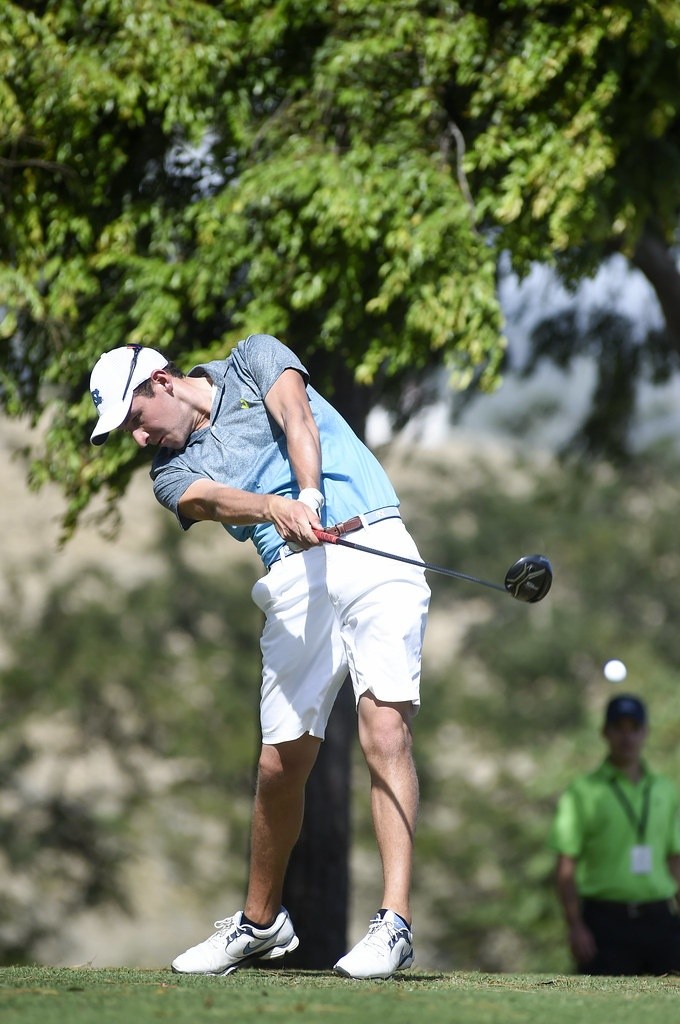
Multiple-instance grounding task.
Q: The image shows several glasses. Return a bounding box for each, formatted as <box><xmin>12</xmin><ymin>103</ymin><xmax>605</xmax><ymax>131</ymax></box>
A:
<box><xmin>121</xmin><ymin>342</ymin><xmax>142</xmax><ymax>401</ymax></box>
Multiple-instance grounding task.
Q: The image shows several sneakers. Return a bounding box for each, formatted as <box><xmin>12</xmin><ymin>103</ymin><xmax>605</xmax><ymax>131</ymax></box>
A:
<box><xmin>170</xmin><ymin>905</ymin><xmax>299</xmax><ymax>976</ymax></box>
<box><xmin>332</xmin><ymin>912</ymin><xmax>415</xmax><ymax>981</ymax></box>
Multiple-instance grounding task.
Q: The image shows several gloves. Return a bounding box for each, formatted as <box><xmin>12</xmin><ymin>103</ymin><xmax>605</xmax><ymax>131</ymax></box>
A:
<box><xmin>288</xmin><ymin>488</ymin><xmax>326</xmax><ymax>553</ymax></box>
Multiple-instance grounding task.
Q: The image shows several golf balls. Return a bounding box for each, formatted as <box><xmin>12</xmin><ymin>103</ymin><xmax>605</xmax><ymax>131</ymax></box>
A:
<box><xmin>604</xmin><ymin>659</ymin><xmax>628</xmax><ymax>684</ymax></box>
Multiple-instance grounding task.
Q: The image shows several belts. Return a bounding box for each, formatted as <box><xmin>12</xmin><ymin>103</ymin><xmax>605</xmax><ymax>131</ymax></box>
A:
<box><xmin>273</xmin><ymin>505</ymin><xmax>401</xmax><ymax>563</ymax></box>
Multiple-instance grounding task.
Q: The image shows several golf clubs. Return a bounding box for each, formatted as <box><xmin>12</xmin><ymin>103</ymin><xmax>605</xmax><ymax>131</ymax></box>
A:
<box><xmin>312</xmin><ymin>527</ymin><xmax>556</xmax><ymax>605</ymax></box>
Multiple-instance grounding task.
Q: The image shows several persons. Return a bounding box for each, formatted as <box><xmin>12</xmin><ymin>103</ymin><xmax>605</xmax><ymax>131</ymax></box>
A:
<box><xmin>544</xmin><ymin>695</ymin><xmax>680</xmax><ymax>978</ymax></box>
<box><xmin>89</xmin><ymin>334</ymin><xmax>432</xmax><ymax>980</ymax></box>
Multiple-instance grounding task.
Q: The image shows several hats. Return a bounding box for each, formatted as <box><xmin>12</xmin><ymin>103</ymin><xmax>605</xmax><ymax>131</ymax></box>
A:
<box><xmin>605</xmin><ymin>697</ymin><xmax>645</xmax><ymax>725</ymax></box>
<box><xmin>90</xmin><ymin>345</ymin><xmax>168</xmax><ymax>448</ymax></box>
